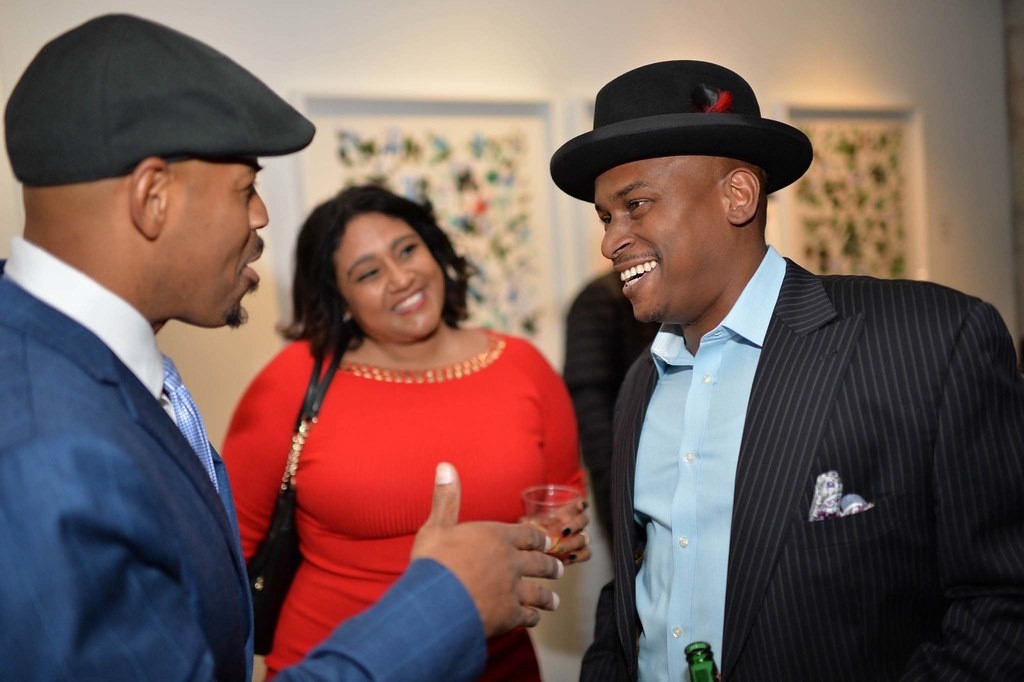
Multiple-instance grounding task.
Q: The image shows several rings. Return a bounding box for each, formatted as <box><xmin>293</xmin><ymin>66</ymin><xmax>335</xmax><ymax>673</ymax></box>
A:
<box><xmin>578</xmin><ymin>532</ymin><xmax>591</xmax><ymax>548</ymax></box>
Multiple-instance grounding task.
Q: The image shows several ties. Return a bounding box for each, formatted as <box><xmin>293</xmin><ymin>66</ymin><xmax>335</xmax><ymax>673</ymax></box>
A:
<box><xmin>160</xmin><ymin>352</ymin><xmax>218</xmax><ymax>497</ymax></box>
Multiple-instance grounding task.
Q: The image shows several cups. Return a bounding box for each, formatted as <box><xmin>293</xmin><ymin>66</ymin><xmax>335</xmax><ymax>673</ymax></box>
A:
<box><xmin>520</xmin><ymin>484</ymin><xmax>582</xmax><ymax>568</ymax></box>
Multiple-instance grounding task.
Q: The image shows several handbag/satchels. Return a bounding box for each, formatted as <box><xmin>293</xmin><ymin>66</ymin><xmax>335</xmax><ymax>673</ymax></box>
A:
<box><xmin>247</xmin><ymin>334</ymin><xmax>352</xmax><ymax>656</ymax></box>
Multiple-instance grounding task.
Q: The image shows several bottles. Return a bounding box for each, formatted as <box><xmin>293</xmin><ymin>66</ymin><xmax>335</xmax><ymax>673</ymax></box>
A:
<box><xmin>684</xmin><ymin>640</ymin><xmax>720</xmax><ymax>682</ymax></box>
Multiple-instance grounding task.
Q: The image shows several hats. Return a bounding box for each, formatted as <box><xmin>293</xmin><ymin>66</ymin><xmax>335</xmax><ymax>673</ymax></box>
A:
<box><xmin>550</xmin><ymin>59</ymin><xmax>814</xmax><ymax>196</ymax></box>
<box><xmin>3</xmin><ymin>11</ymin><xmax>315</xmax><ymax>185</ymax></box>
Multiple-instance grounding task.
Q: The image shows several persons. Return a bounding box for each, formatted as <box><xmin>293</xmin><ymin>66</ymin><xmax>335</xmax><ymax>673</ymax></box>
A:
<box><xmin>219</xmin><ymin>185</ymin><xmax>590</xmax><ymax>682</ymax></box>
<box><xmin>547</xmin><ymin>57</ymin><xmax>1023</xmax><ymax>681</ymax></box>
<box><xmin>3</xmin><ymin>12</ymin><xmax>564</xmax><ymax>682</ymax></box>
